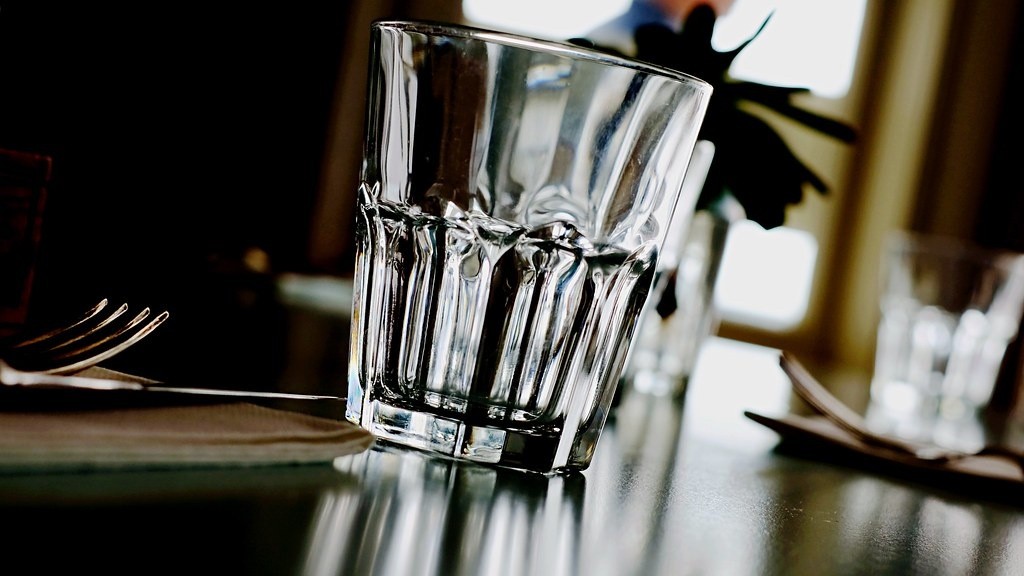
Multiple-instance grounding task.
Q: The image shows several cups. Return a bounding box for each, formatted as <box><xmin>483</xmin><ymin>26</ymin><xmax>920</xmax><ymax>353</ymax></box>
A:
<box><xmin>864</xmin><ymin>236</ymin><xmax>1023</xmax><ymax>458</ymax></box>
<box><xmin>342</xmin><ymin>19</ymin><xmax>715</xmax><ymax>476</ymax></box>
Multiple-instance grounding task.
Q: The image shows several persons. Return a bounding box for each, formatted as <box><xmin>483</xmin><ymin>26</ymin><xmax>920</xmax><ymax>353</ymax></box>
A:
<box><xmin>561</xmin><ymin>0</ymin><xmax>741</xmax><ymax>196</ymax></box>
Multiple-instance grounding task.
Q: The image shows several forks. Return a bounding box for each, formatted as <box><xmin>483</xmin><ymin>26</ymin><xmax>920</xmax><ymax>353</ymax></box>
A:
<box><xmin>0</xmin><ymin>298</ymin><xmax>169</xmax><ymax>376</ymax></box>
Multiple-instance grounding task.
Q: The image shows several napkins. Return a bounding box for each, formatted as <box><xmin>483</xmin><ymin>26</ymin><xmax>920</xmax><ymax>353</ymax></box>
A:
<box><xmin>0</xmin><ymin>367</ymin><xmax>376</xmax><ymax>465</ymax></box>
<box><xmin>742</xmin><ymin>409</ymin><xmax>1024</xmax><ymax>507</ymax></box>
